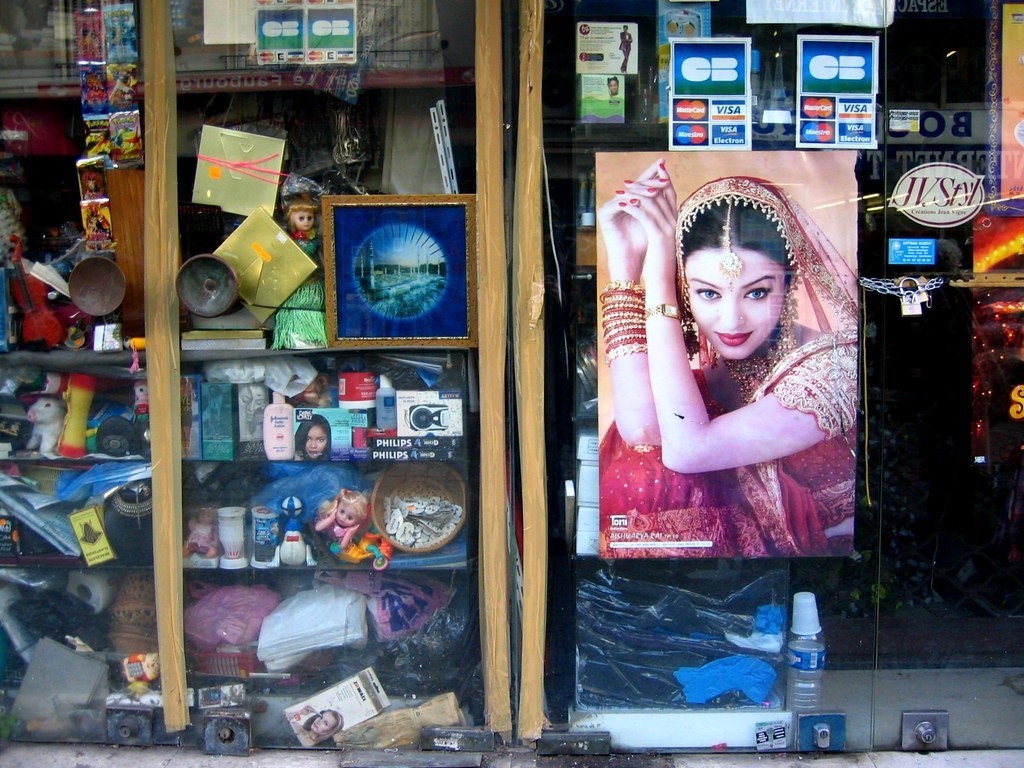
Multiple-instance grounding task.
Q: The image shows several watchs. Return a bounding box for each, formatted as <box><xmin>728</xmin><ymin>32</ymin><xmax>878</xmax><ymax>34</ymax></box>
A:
<box><xmin>645</xmin><ymin>304</ymin><xmax>679</xmax><ymax>322</ymax></box>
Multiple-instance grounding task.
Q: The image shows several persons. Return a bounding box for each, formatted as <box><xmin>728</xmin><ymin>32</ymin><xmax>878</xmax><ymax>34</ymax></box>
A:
<box><xmin>206</xmin><ymin>386</ymin><xmax>225</xmax><ymax>425</ymax></box>
<box><xmin>296</xmin><ymin>413</ymin><xmax>332</xmax><ymax>462</ymax></box>
<box><xmin>607</xmin><ymin>76</ymin><xmax>619</xmax><ymax>95</ymax></box>
<box><xmin>316</xmin><ymin>490</ymin><xmax>369</xmax><ymax>555</ymax></box>
<box><xmin>238</xmin><ymin>384</ymin><xmax>266</xmax><ymax>442</ymax></box>
<box><xmin>288</xmin><ymin>708</ymin><xmax>342</xmax><ymax>747</ymax></box>
<box><xmin>619</xmin><ymin>25</ymin><xmax>633</xmax><ymax>73</ymax></box>
<box><xmin>184</xmin><ymin>503</ymin><xmax>218</xmax><ymax>557</ymax></box>
<box><xmin>599</xmin><ymin>160</ymin><xmax>859</xmax><ymax>555</ymax></box>
<box><xmin>273</xmin><ymin>196</ymin><xmax>328</xmax><ymax>349</ymax></box>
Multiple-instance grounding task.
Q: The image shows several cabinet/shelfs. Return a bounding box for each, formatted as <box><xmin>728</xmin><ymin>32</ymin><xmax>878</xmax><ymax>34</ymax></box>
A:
<box><xmin>517</xmin><ymin>0</ymin><xmax>1024</xmax><ymax>755</ymax></box>
<box><xmin>0</xmin><ymin>0</ymin><xmax>519</xmax><ymax>751</ymax></box>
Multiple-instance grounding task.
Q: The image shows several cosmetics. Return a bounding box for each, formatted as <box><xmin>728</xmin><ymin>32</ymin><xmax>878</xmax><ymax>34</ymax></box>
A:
<box><xmin>263</xmin><ymin>390</ymin><xmax>295</xmax><ymax>460</ymax></box>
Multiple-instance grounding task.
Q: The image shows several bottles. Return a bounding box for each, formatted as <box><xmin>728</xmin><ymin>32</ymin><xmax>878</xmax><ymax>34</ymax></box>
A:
<box><xmin>375</xmin><ymin>374</ymin><xmax>397</xmax><ymax>430</ymax></box>
<box><xmin>786</xmin><ymin>630</ymin><xmax>826</xmax><ymax>714</ymax></box>
<box><xmin>262</xmin><ymin>391</ymin><xmax>296</xmax><ymax>461</ymax></box>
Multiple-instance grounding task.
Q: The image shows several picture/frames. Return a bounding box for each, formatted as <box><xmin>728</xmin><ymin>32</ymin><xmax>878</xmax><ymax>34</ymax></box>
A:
<box><xmin>984</xmin><ymin>0</ymin><xmax>1023</xmax><ymax>216</ymax></box>
<box><xmin>320</xmin><ymin>194</ymin><xmax>479</xmax><ymax>347</ymax></box>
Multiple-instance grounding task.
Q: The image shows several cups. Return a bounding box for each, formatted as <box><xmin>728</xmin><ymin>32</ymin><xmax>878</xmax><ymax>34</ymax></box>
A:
<box><xmin>790</xmin><ymin>592</ymin><xmax>822</xmax><ymax>635</ymax></box>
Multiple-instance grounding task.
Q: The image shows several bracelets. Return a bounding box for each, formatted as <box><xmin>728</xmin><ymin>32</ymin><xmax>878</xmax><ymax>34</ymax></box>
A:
<box><xmin>599</xmin><ymin>281</ymin><xmax>648</xmax><ymax>367</ymax></box>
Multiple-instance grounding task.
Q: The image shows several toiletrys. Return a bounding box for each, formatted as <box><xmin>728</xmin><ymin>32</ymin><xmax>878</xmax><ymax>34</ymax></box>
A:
<box><xmin>375</xmin><ymin>375</ymin><xmax>397</xmax><ymax>432</ymax></box>
<box><xmin>263</xmin><ymin>391</ymin><xmax>295</xmax><ymax>459</ymax></box>
